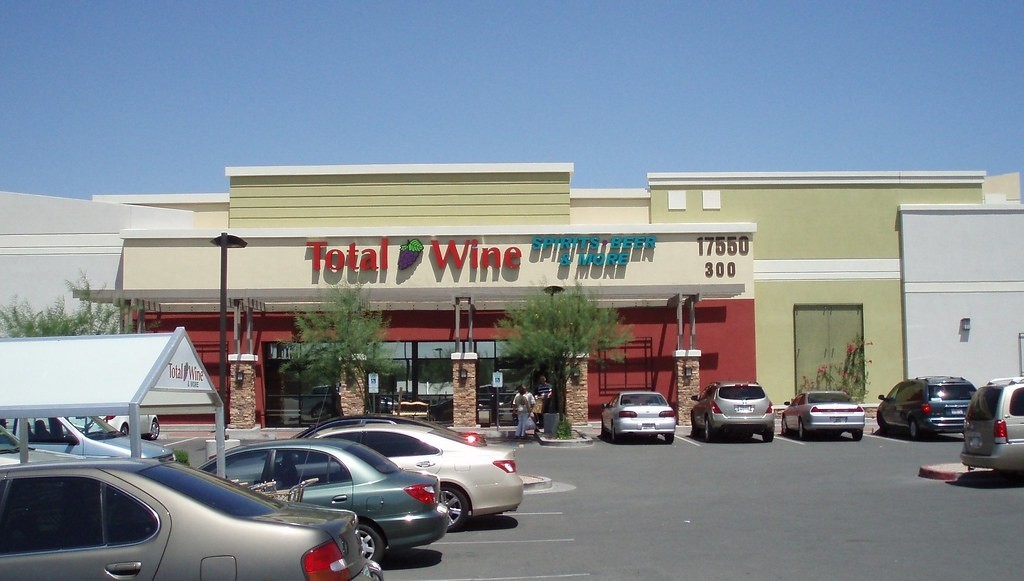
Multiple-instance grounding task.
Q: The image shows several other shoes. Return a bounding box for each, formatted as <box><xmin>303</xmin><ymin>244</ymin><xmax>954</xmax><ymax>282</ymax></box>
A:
<box><xmin>520</xmin><ymin>436</ymin><xmax>525</xmax><ymax>439</ymax></box>
<box><xmin>515</xmin><ymin>436</ymin><xmax>519</xmax><ymax>439</ymax></box>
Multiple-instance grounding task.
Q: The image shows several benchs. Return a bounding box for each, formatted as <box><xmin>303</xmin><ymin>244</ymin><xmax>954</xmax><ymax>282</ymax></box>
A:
<box><xmin>392</xmin><ymin>400</ymin><xmax>429</xmax><ymax>420</ymax></box>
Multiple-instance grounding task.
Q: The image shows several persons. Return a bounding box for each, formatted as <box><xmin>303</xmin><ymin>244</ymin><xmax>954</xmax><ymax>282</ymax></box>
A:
<box><xmin>498</xmin><ymin>376</ymin><xmax>553</xmax><ymax>439</ymax></box>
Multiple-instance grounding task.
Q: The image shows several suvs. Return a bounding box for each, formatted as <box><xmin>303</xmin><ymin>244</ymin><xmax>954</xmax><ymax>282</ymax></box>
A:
<box><xmin>689</xmin><ymin>380</ymin><xmax>775</xmax><ymax>443</ymax></box>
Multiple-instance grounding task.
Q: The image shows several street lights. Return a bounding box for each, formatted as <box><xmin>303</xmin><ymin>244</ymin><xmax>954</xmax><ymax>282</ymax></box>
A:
<box><xmin>210</xmin><ymin>231</ymin><xmax>247</xmax><ymax>481</ymax></box>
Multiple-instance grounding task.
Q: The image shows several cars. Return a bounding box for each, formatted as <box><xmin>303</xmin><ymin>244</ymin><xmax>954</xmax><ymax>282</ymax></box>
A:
<box><xmin>876</xmin><ymin>375</ymin><xmax>979</xmax><ymax>441</ymax></box>
<box><xmin>0</xmin><ymin>456</ymin><xmax>374</xmax><ymax>581</ymax></box>
<box><xmin>959</xmin><ymin>376</ymin><xmax>1024</xmax><ymax>472</ymax></box>
<box><xmin>600</xmin><ymin>390</ymin><xmax>677</xmax><ymax>444</ymax></box>
<box><xmin>781</xmin><ymin>391</ymin><xmax>866</xmax><ymax>441</ymax></box>
<box><xmin>0</xmin><ymin>413</ymin><xmax>177</xmax><ymax>465</ymax></box>
<box><xmin>289</xmin><ymin>413</ymin><xmax>489</xmax><ymax>449</ymax></box>
<box><xmin>0</xmin><ymin>423</ymin><xmax>85</xmax><ymax>469</ymax></box>
<box><xmin>192</xmin><ymin>437</ymin><xmax>450</xmax><ymax>566</ymax></box>
<box><xmin>301</xmin><ymin>423</ymin><xmax>527</xmax><ymax>531</ymax></box>
<box><xmin>64</xmin><ymin>414</ymin><xmax>160</xmax><ymax>440</ymax></box>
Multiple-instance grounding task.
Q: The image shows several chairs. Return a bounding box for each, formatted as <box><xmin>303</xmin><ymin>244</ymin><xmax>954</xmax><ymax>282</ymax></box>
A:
<box><xmin>34</xmin><ymin>420</ymin><xmax>52</xmax><ymax>442</ymax></box>
<box><xmin>275</xmin><ymin>456</ymin><xmax>298</xmax><ymax>490</ymax></box>
<box><xmin>27</xmin><ymin>422</ymin><xmax>36</xmax><ymax>441</ymax></box>
<box><xmin>623</xmin><ymin>400</ymin><xmax>631</xmax><ymax>404</ymax></box>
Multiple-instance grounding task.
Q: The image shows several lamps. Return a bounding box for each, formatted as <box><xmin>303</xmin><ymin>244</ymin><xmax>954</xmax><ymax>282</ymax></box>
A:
<box><xmin>236</xmin><ymin>371</ymin><xmax>244</xmax><ymax>381</ymax></box>
<box><xmin>460</xmin><ymin>368</ymin><xmax>468</xmax><ymax>378</ymax></box>
<box><xmin>685</xmin><ymin>368</ymin><xmax>692</xmax><ymax>376</ymax></box>
<box><xmin>961</xmin><ymin>318</ymin><xmax>971</xmax><ymax>330</ymax></box>
<box><xmin>573</xmin><ymin>370</ymin><xmax>581</xmax><ymax>377</ymax></box>
